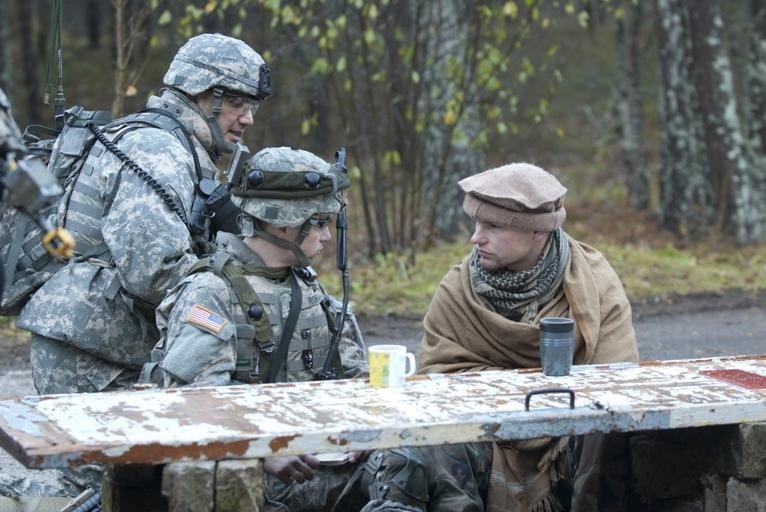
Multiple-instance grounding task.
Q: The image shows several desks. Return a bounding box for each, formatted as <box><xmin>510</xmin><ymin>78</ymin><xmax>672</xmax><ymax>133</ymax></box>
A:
<box><xmin>1</xmin><ymin>340</ymin><xmax>765</xmax><ymax>511</ymax></box>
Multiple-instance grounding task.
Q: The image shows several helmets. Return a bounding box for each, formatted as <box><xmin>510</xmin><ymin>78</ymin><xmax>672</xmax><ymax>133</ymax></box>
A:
<box><xmin>230</xmin><ymin>146</ymin><xmax>342</xmax><ymax>229</ymax></box>
<box><xmin>164</xmin><ymin>33</ymin><xmax>272</xmax><ymax>103</ymax></box>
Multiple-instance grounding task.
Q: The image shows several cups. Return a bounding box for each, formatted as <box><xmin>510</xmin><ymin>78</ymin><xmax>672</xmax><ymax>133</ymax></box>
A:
<box><xmin>368</xmin><ymin>342</ymin><xmax>417</xmax><ymax>391</ymax></box>
<box><xmin>538</xmin><ymin>316</ymin><xmax>577</xmax><ymax>376</ymax></box>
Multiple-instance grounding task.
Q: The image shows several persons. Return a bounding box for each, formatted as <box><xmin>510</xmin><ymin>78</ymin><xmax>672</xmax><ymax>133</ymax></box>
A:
<box><xmin>412</xmin><ymin>159</ymin><xmax>640</xmax><ymax>511</ymax></box>
<box><xmin>0</xmin><ymin>31</ymin><xmax>273</xmax><ymax>512</ymax></box>
<box><xmin>137</xmin><ymin>144</ymin><xmax>430</xmax><ymax>511</ymax></box>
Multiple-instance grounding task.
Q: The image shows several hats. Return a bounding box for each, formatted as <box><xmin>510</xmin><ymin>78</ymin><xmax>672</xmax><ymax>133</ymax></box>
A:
<box><xmin>457</xmin><ymin>162</ymin><xmax>568</xmax><ymax>232</ymax></box>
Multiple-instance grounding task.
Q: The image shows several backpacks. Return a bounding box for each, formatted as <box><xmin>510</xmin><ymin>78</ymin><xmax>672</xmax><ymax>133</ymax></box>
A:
<box><xmin>1</xmin><ymin>111</ymin><xmax>200</xmax><ymax>316</ymax></box>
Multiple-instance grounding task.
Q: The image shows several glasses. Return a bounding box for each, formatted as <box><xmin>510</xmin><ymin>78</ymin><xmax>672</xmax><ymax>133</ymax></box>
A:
<box><xmin>222</xmin><ymin>94</ymin><xmax>261</xmax><ymax>117</ymax></box>
<box><xmin>309</xmin><ymin>214</ymin><xmax>334</xmax><ymax>230</ymax></box>
